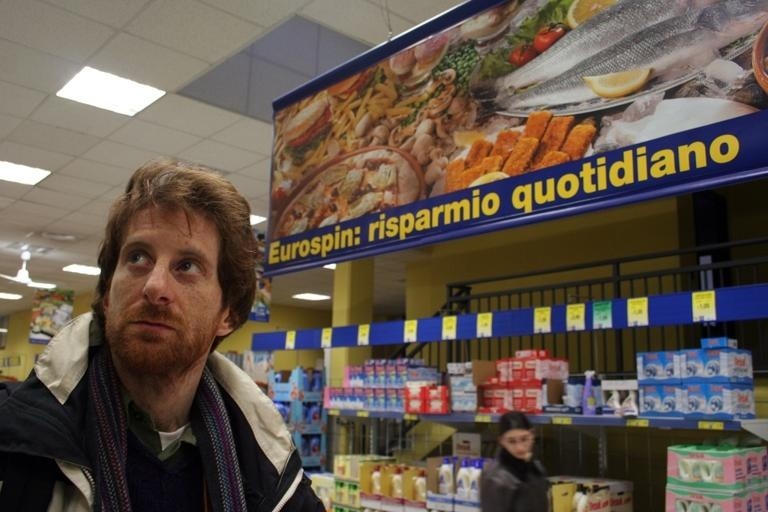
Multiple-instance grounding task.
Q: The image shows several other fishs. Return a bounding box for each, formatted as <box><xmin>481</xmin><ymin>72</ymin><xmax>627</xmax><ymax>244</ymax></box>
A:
<box><xmin>471</xmin><ymin>0</ymin><xmax>768</xmax><ymax>120</ymax></box>
<box><xmin>471</xmin><ymin>0</ymin><xmax>729</xmax><ymax>103</ymax></box>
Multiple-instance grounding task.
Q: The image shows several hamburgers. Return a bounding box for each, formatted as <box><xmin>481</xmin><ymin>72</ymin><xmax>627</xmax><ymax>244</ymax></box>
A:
<box><xmin>414</xmin><ymin>31</ymin><xmax>450</xmax><ymax>69</ymax></box>
<box><xmin>280</xmin><ymin>100</ymin><xmax>334</xmax><ymax>169</ymax></box>
<box><xmin>389</xmin><ymin>48</ymin><xmax>414</xmax><ymax>75</ymax></box>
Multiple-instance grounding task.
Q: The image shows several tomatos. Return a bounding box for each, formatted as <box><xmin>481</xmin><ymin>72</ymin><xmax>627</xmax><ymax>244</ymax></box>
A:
<box><xmin>511</xmin><ymin>45</ymin><xmax>536</xmax><ymax>68</ymax></box>
<box><xmin>533</xmin><ymin>23</ymin><xmax>566</xmax><ymax>54</ymax></box>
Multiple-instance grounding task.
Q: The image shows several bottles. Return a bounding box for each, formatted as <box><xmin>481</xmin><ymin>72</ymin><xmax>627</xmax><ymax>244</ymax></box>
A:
<box><xmin>302</xmin><ymin>401</ymin><xmax>322</xmax><ymax>425</ymax></box>
<box><xmin>301</xmin><ymin>434</ymin><xmax>319</xmax><ymax>456</ymax></box>
<box><xmin>371</xmin><ymin>461</ymin><xmax>426</xmax><ymax>504</ymax></box>
<box><xmin>302</xmin><ymin>369</ymin><xmax>322</xmax><ymax>393</ymax></box>
<box><xmin>437</xmin><ymin>454</ymin><xmax>486</xmax><ymax>502</ymax></box>
<box><xmin>273</xmin><ymin>400</ymin><xmax>291</xmax><ymax>423</ymax></box>
<box><xmin>570</xmin><ymin>483</ymin><xmax>599</xmax><ymax>511</ymax></box>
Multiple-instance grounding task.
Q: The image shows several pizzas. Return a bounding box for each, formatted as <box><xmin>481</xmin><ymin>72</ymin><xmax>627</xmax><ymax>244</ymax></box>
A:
<box><xmin>271</xmin><ymin>149</ymin><xmax>425</xmax><ymax>241</ymax></box>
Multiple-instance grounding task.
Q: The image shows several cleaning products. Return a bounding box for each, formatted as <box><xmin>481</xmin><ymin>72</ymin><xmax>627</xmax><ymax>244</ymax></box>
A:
<box><xmin>582</xmin><ymin>369</ymin><xmax>597</xmax><ymax>416</ymax></box>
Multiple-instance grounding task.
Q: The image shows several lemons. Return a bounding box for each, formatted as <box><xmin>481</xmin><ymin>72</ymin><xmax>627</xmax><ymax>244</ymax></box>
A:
<box><xmin>567</xmin><ymin>1</ymin><xmax>618</xmax><ymax>29</ymax></box>
<box><xmin>583</xmin><ymin>69</ymin><xmax>653</xmax><ymax>99</ymax></box>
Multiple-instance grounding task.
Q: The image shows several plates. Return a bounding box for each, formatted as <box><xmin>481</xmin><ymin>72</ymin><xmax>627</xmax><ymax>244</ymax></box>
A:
<box><xmin>470</xmin><ymin>34</ymin><xmax>758</xmax><ymax>116</ymax></box>
<box><xmin>273</xmin><ymin>146</ymin><xmax>424</xmax><ymax>243</ymax></box>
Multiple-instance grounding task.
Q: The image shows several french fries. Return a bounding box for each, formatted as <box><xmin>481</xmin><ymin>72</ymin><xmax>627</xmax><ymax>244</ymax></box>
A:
<box><xmin>304</xmin><ymin>78</ymin><xmax>414</xmax><ymax>160</ymax></box>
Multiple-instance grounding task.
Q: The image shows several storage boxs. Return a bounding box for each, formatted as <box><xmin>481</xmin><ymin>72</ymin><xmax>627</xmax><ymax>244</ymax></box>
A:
<box><xmin>309</xmin><ymin>440</ymin><xmax>639</xmax><ymax>511</ymax></box>
<box><xmin>635</xmin><ymin>337</ymin><xmax>756</xmax><ymax>417</ymax></box>
<box><xmin>267</xmin><ymin>367</ymin><xmax>323</xmax><ymax>467</ymax></box>
<box><xmin>662</xmin><ymin>441</ymin><xmax>768</xmax><ymax>510</ymax></box>
<box><xmin>324</xmin><ymin>348</ymin><xmax>568</xmax><ymax>414</ymax></box>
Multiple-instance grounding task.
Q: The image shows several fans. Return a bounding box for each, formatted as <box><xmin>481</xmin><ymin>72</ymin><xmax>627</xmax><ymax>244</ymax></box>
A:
<box><xmin>0</xmin><ymin>249</ymin><xmax>34</xmax><ymax>288</ymax></box>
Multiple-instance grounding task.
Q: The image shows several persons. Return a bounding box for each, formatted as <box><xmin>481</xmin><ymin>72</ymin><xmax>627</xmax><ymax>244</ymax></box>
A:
<box><xmin>478</xmin><ymin>412</ymin><xmax>549</xmax><ymax>512</ymax></box>
<box><xmin>0</xmin><ymin>156</ymin><xmax>330</xmax><ymax>512</ymax></box>
